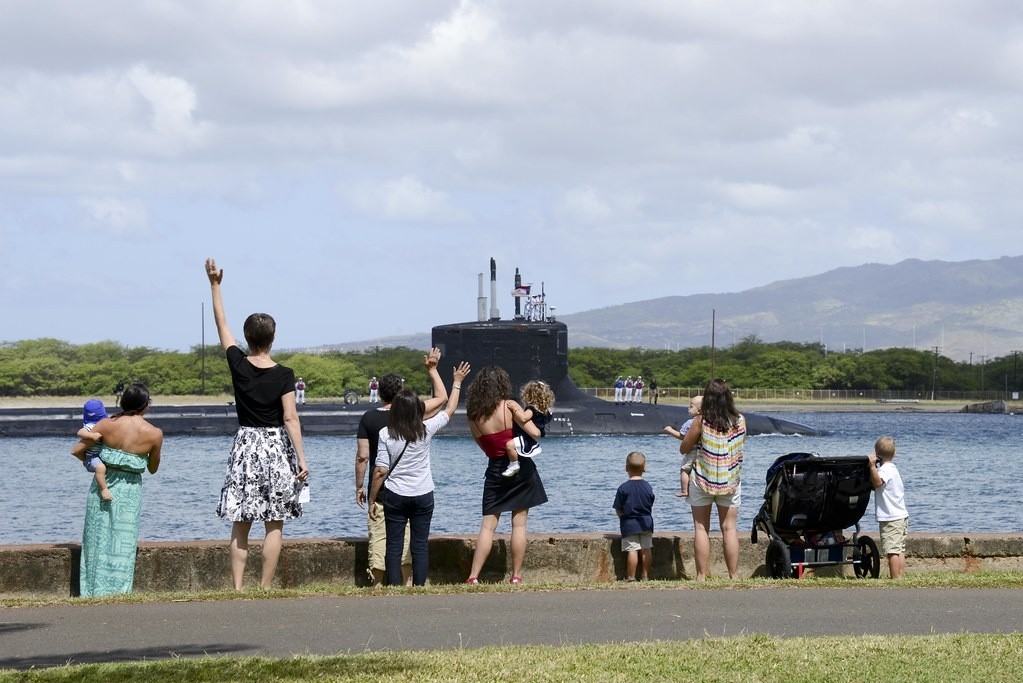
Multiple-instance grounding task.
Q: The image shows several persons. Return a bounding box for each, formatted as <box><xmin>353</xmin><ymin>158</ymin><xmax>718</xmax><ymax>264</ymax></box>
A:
<box><xmin>633</xmin><ymin>376</ymin><xmax>644</xmax><ymax>403</ymax></box>
<box><xmin>77</xmin><ymin>399</ymin><xmax>114</xmax><ymax>501</ymax></box>
<box><xmin>294</xmin><ymin>377</ymin><xmax>306</xmax><ymax>405</ymax></box>
<box><xmin>466</xmin><ymin>365</ymin><xmax>548</xmax><ymax>584</ymax></box>
<box><xmin>501</xmin><ymin>378</ymin><xmax>556</xmax><ymax>476</ymax></box>
<box><xmin>369</xmin><ymin>376</ymin><xmax>380</xmax><ymax>403</ymax></box>
<box><xmin>203</xmin><ymin>258</ymin><xmax>311</xmax><ymax>594</ymax></box>
<box><xmin>612</xmin><ymin>452</ymin><xmax>655</xmax><ymax>581</ymax></box>
<box><xmin>615</xmin><ymin>375</ymin><xmax>623</xmax><ymax>401</ymax></box>
<box><xmin>680</xmin><ymin>377</ymin><xmax>746</xmax><ymax>581</ymax></box>
<box><xmin>624</xmin><ymin>376</ymin><xmax>633</xmax><ymax>401</ymax></box>
<box><xmin>367</xmin><ymin>362</ymin><xmax>471</xmax><ymax>586</ymax></box>
<box><xmin>867</xmin><ymin>437</ymin><xmax>907</xmax><ymax>580</ymax></box>
<box><xmin>71</xmin><ymin>386</ymin><xmax>164</xmax><ymax>597</ymax></box>
<box><xmin>354</xmin><ymin>346</ymin><xmax>449</xmax><ymax>589</ymax></box>
<box><xmin>648</xmin><ymin>378</ymin><xmax>659</xmax><ymax>404</ymax></box>
<box><xmin>663</xmin><ymin>394</ymin><xmax>704</xmax><ymax>497</ymax></box>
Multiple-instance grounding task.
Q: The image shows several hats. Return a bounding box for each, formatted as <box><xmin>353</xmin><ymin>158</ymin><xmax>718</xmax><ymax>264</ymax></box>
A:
<box><xmin>373</xmin><ymin>377</ymin><xmax>376</xmax><ymax>380</ymax></box>
<box><xmin>638</xmin><ymin>376</ymin><xmax>641</xmax><ymax>378</ymax></box>
<box><xmin>83</xmin><ymin>399</ymin><xmax>107</xmax><ymax>424</ymax></box>
<box><xmin>299</xmin><ymin>378</ymin><xmax>302</xmax><ymax>380</ymax></box>
<box><xmin>628</xmin><ymin>376</ymin><xmax>631</xmax><ymax>378</ymax></box>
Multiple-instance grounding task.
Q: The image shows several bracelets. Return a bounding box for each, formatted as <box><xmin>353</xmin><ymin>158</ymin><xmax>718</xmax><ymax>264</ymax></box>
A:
<box><xmin>357</xmin><ymin>487</ymin><xmax>364</xmax><ymax>493</ymax></box>
<box><xmin>452</xmin><ymin>385</ymin><xmax>461</xmax><ymax>389</ymax></box>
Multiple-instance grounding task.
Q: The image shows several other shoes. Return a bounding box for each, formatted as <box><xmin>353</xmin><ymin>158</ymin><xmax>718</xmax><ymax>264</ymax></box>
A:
<box><xmin>510</xmin><ymin>575</ymin><xmax>521</xmax><ymax>585</ymax></box>
<box><xmin>466</xmin><ymin>578</ymin><xmax>477</xmax><ymax>585</ymax></box>
<box><xmin>502</xmin><ymin>465</ymin><xmax>520</xmax><ymax>477</ymax></box>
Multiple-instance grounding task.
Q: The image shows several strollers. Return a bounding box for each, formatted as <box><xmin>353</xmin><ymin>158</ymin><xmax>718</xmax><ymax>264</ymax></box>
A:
<box><xmin>751</xmin><ymin>451</ymin><xmax>883</xmax><ymax>582</ymax></box>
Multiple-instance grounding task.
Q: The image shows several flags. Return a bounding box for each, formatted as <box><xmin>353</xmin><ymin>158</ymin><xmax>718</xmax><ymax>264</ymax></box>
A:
<box><xmin>511</xmin><ymin>286</ymin><xmax>530</xmax><ymax>297</ymax></box>
